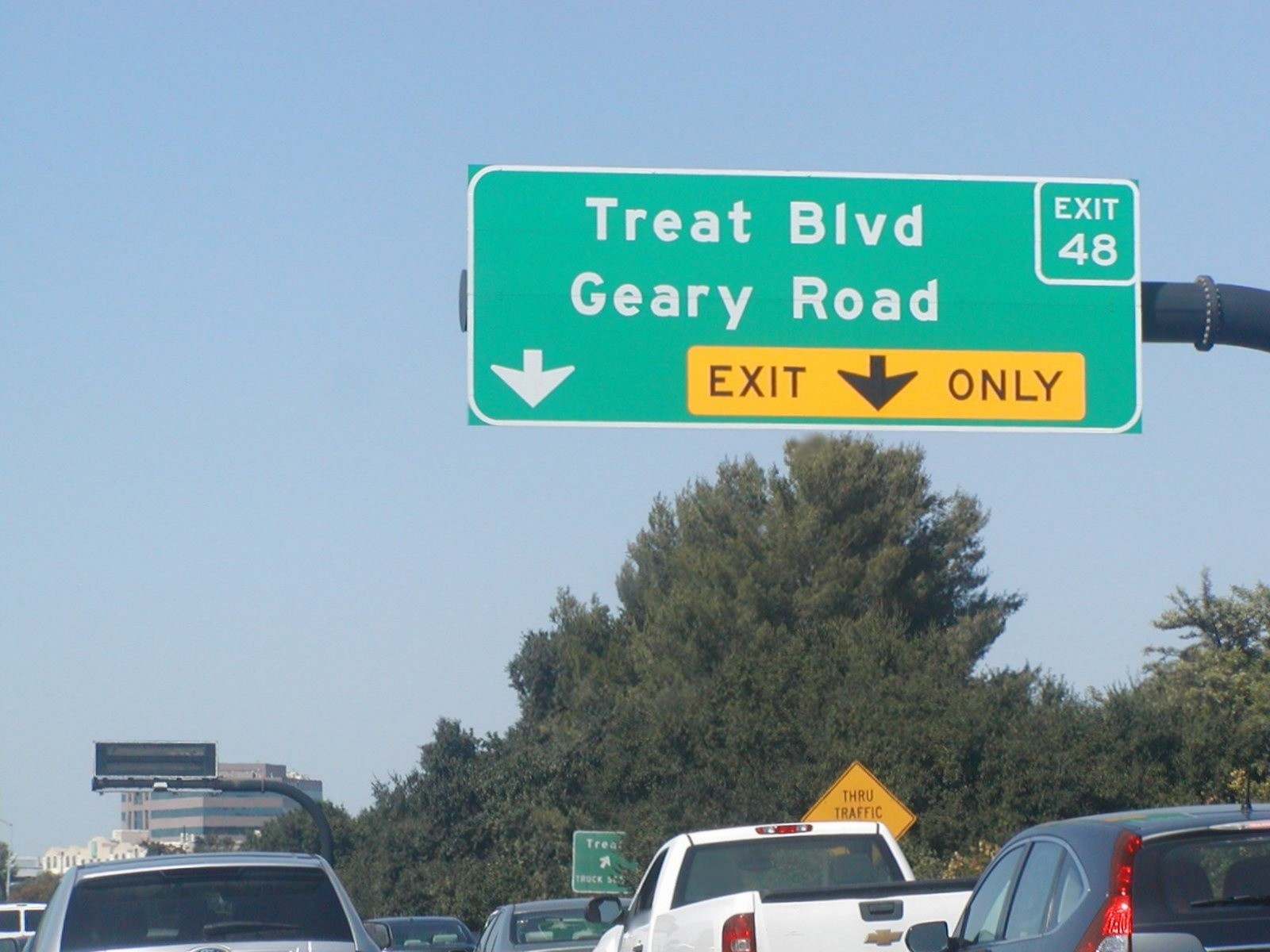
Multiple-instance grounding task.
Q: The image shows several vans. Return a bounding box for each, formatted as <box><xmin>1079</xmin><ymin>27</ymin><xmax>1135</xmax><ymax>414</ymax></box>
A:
<box><xmin>0</xmin><ymin>903</ymin><xmax>47</xmax><ymax>952</ymax></box>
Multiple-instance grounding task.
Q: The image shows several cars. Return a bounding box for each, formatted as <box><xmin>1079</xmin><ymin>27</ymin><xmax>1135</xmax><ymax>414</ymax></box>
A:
<box><xmin>473</xmin><ymin>897</ymin><xmax>633</xmax><ymax>952</ymax></box>
<box><xmin>0</xmin><ymin>851</ymin><xmax>382</xmax><ymax>952</ymax></box>
<box><xmin>363</xmin><ymin>915</ymin><xmax>478</xmax><ymax>952</ymax></box>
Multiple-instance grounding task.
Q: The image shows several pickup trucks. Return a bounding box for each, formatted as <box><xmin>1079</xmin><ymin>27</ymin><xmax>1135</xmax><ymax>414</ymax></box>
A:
<box><xmin>583</xmin><ymin>821</ymin><xmax>979</xmax><ymax>952</ymax></box>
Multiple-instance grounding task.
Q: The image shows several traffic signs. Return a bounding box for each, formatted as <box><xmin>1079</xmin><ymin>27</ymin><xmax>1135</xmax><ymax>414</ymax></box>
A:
<box><xmin>467</xmin><ymin>161</ymin><xmax>1146</xmax><ymax>439</ymax></box>
<box><xmin>572</xmin><ymin>830</ymin><xmax>635</xmax><ymax>894</ymax></box>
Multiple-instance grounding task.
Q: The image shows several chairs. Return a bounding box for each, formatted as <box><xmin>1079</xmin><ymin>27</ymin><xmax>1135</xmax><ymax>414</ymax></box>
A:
<box><xmin>1224</xmin><ymin>857</ymin><xmax>1270</xmax><ymax>900</ymax></box>
<box><xmin>525</xmin><ymin>931</ymin><xmax>553</xmax><ymax>943</ymax></box>
<box><xmin>432</xmin><ymin>934</ymin><xmax>458</xmax><ymax>945</ymax></box>
<box><xmin>572</xmin><ymin>930</ymin><xmax>595</xmax><ymax>941</ymax></box>
<box><xmin>829</xmin><ymin>853</ymin><xmax>874</xmax><ymax>885</ymax></box>
<box><xmin>1164</xmin><ymin>862</ymin><xmax>1213</xmax><ymax>907</ymax></box>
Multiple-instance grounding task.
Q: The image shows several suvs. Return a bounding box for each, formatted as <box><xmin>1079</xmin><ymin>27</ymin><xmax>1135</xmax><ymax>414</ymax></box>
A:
<box><xmin>904</xmin><ymin>801</ymin><xmax>1270</xmax><ymax>952</ymax></box>
